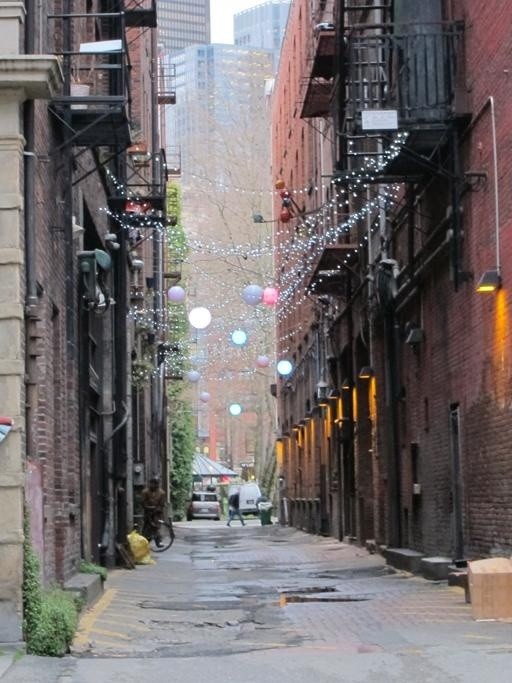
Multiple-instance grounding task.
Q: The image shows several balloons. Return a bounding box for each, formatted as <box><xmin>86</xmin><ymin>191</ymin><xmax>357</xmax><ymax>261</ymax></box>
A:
<box><xmin>232</xmin><ymin>331</ymin><xmax>247</xmax><ymax>344</ymax></box>
<box><xmin>275</xmin><ymin>179</ymin><xmax>291</xmax><ymax>223</ymax></box>
<box><xmin>242</xmin><ymin>285</ymin><xmax>278</xmax><ymax>305</ymax></box>
<box><xmin>229</xmin><ymin>405</ymin><xmax>241</xmax><ymax>415</ymax></box>
<box><xmin>200</xmin><ymin>392</ymin><xmax>210</xmax><ymax>403</ymax></box>
<box><xmin>168</xmin><ymin>286</ymin><xmax>186</xmax><ymax>303</ymax></box>
<box><xmin>188</xmin><ymin>307</ymin><xmax>211</xmax><ymax>329</ymax></box>
<box><xmin>258</xmin><ymin>356</ymin><xmax>269</xmax><ymax>365</ymax></box>
<box><xmin>278</xmin><ymin>361</ymin><xmax>292</xmax><ymax>375</ymax></box>
<box><xmin>188</xmin><ymin>371</ymin><xmax>200</xmax><ymax>382</ymax></box>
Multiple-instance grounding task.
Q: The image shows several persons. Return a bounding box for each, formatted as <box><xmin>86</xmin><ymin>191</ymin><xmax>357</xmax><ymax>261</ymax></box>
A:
<box><xmin>142</xmin><ymin>476</ymin><xmax>166</xmax><ymax>547</ymax></box>
<box><xmin>226</xmin><ymin>486</ymin><xmax>247</xmax><ymax>527</ymax></box>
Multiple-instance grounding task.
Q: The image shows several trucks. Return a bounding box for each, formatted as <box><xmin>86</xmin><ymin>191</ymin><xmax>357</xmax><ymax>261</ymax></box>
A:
<box><xmin>227</xmin><ymin>482</ymin><xmax>263</xmax><ymax>519</ymax></box>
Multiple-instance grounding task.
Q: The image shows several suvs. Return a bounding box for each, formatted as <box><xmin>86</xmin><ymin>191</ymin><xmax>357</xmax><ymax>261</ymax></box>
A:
<box><xmin>184</xmin><ymin>491</ymin><xmax>223</xmax><ymax>521</ymax></box>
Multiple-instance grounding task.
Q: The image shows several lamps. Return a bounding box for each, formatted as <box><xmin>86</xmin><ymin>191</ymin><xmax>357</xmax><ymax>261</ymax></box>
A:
<box><xmin>327</xmin><ymin>390</ymin><xmax>343</xmax><ymax>400</ymax></box>
<box><xmin>276</xmin><ymin>397</ymin><xmax>328</xmax><ymax>445</ymax></box>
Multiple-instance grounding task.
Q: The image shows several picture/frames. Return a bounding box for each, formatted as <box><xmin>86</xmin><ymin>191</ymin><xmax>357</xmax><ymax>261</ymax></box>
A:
<box><xmin>473</xmin><ymin>270</ymin><xmax>501</xmax><ymax>294</ymax></box>
<box><xmin>340</xmin><ymin>377</ymin><xmax>355</xmax><ymax>392</ymax></box>
<box><xmin>358</xmin><ymin>366</ymin><xmax>375</xmax><ymax>381</ymax></box>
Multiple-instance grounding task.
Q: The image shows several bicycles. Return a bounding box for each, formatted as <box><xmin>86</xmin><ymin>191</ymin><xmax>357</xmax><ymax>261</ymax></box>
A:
<box><xmin>132</xmin><ymin>503</ymin><xmax>176</xmax><ymax>553</ymax></box>
